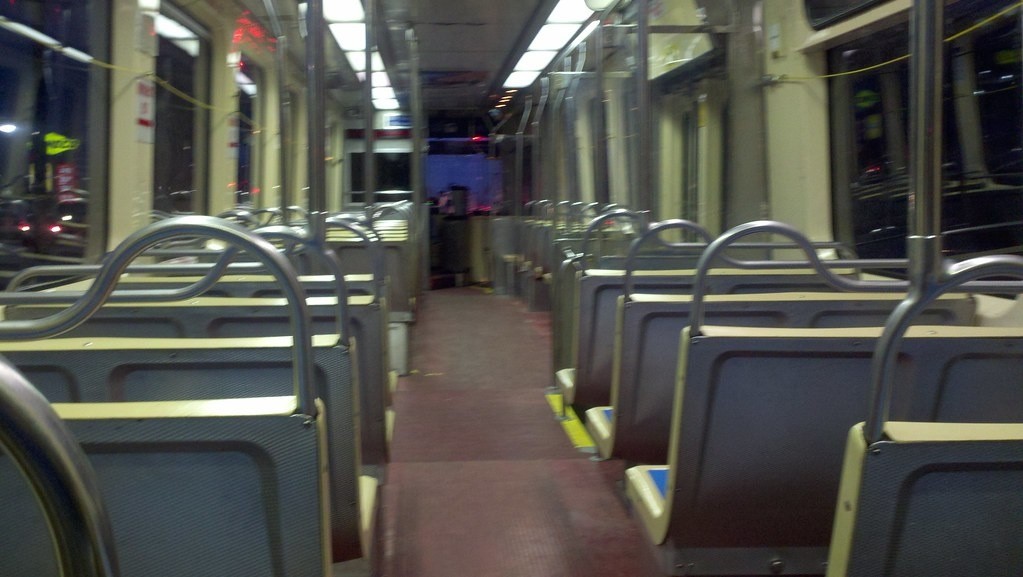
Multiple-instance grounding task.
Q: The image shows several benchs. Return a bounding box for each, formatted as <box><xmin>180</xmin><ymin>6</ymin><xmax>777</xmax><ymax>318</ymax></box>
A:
<box><xmin>520</xmin><ymin>198</ymin><xmax>1022</xmax><ymax>577</ymax></box>
<box><xmin>0</xmin><ymin>190</ymin><xmax>415</xmax><ymax>576</ymax></box>
